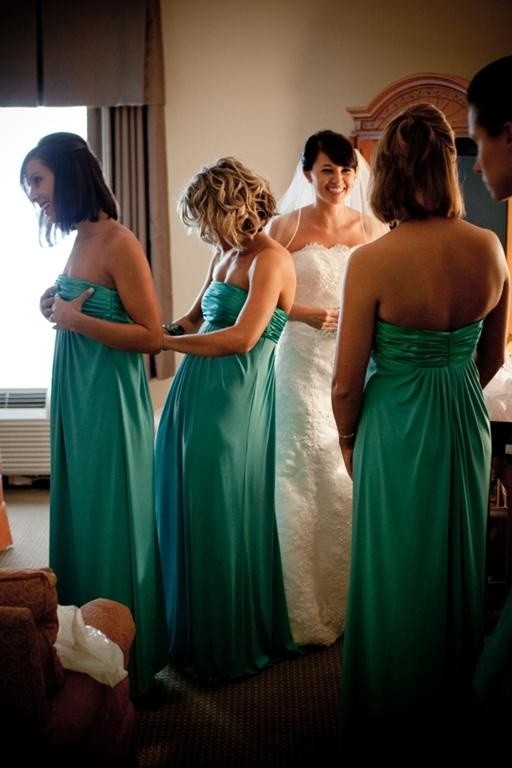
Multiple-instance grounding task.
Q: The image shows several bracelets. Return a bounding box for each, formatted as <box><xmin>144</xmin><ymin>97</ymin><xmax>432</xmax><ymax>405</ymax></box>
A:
<box><xmin>162</xmin><ymin>322</ymin><xmax>184</xmax><ymax>335</ymax></box>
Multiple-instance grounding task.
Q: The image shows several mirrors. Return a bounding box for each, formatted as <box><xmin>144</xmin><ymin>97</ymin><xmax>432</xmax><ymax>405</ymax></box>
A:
<box><xmin>340</xmin><ymin>68</ymin><xmax>511</xmax><ymax>362</ymax></box>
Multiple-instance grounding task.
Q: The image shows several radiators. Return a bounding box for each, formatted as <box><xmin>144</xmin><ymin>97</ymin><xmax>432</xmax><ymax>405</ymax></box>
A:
<box><xmin>0</xmin><ymin>388</ymin><xmax>53</xmax><ymax>491</ymax></box>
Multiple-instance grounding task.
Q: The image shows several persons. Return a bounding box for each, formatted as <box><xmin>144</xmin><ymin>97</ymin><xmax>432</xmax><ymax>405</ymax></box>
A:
<box><xmin>155</xmin><ymin>158</ymin><xmax>304</xmax><ymax>689</ymax></box>
<box><xmin>333</xmin><ymin>103</ymin><xmax>511</xmax><ymax>727</ymax></box>
<box><xmin>22</xmin><ymin>133</ymin><xmax>165</xmax><ymax>695</ymax></box>
<box><xmin>264</xmin><ymin>129</ymin><xmax>392</xmax><ymax>649</ymax></box>
<box><xmin>466</xmin><ymin>57</ymin><xmax>511</xmax><ymax>202</ymax></box>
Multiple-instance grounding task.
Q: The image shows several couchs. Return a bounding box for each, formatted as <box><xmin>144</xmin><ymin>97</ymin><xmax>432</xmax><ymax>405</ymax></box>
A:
<box><xmin>2</xmin><ymin>548</ymin><xmax>137</xmax><ymax>767</ymax></box>
